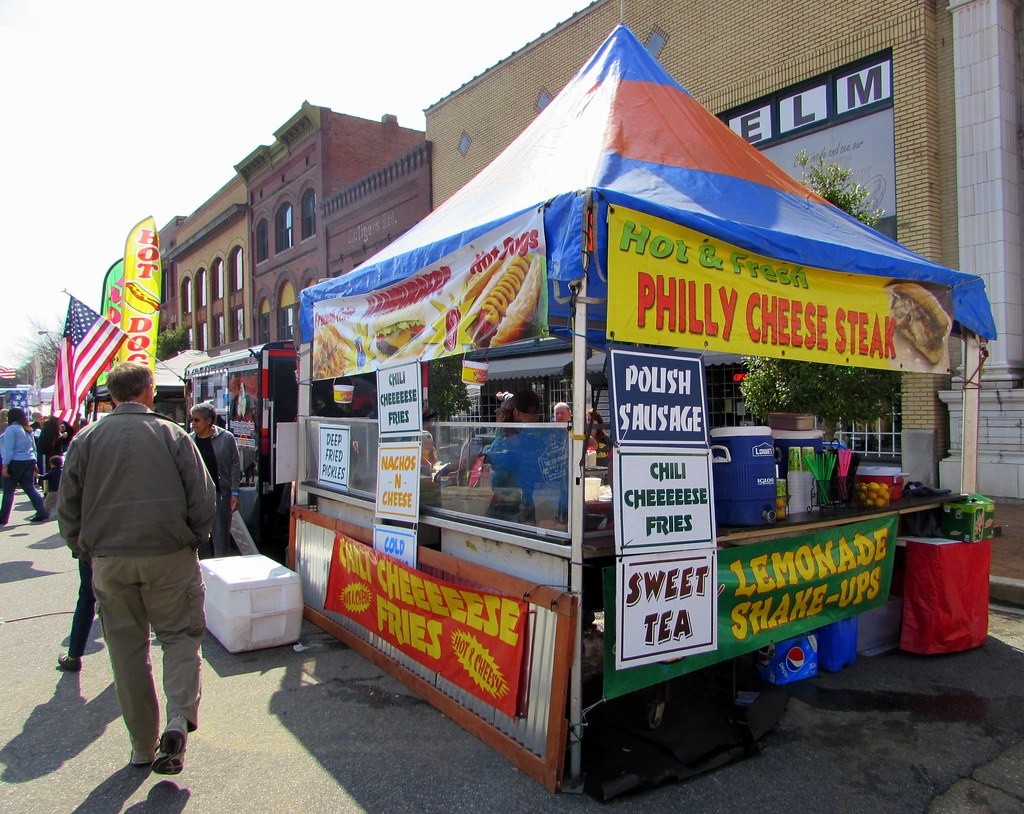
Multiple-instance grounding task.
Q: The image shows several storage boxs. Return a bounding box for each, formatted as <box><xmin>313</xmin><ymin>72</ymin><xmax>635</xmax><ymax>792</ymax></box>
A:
<box><xmin>854</xmin><ymin>465</ymin><xmax>910</xmax><ymax>501</ymax></box>
<box><xmin>938</xmin><ymin>491</ymin><xmax>996</xmax><ymax>545</ymax></box>
<box><xmin>754</xmin><ymin>633</ymin><xmax>820</xmax><ymax>685</ymax></box>
<box><xmin>200</xmin><ymin>553</ymin><xmax>303</xmax><ymax>654</ymax></box>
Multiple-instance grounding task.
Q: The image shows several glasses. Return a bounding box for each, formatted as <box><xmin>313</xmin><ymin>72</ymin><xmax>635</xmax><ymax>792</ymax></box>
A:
<box><xmin>190</xmin><ymin>417</ymin><xmax>200</xmax><ymax>423</ymax></box>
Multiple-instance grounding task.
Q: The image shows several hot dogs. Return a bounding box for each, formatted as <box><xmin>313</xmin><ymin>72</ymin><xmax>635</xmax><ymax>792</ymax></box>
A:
<box><xmin>464</xmin><ymin>247</ymin><xmax>541</xmax><ymax>347</ymax></box>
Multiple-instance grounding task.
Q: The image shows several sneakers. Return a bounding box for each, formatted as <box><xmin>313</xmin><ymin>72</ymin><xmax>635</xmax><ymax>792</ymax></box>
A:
<box><xmin>150</xmin><ymin>716</ymin><xmax>188</xmax><ymax>775</ymax></box>
<box><xmin>58</xmin><ymin>649</ymin><xmax>82</xmax><ymax>671</ymax></box>
<box><xmin>131</xmin><ymin>736</ymin><xmax>161</xmax><ymax>764</ymax></box>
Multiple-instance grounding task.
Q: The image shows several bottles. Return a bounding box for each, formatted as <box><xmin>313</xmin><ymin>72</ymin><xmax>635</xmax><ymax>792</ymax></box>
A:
<box><xmin>584</xmin><ymin>444</ymin><xmax>596</xmax><ymax>471</ymax></box>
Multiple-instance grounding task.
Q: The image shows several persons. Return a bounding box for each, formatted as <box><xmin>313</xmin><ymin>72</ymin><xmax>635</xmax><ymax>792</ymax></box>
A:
<box><xmin>188</xmin><ymin>403</ymin><xmax>241</xmax><ymax>560</ymax></box>
<box><xmin>57</xmin><ymin>361</ymin><xmax>217</xmax><ymax>775</ymax></box>
<box><xmin>0</xmin><ymin>409</ymin><xmax>88</xmax><ymax>527</ymax></box>
<box><xmin>228</xmin><ymin>379</ymin><xmax>257</xmax><ymax>438</ymax></box>
<box><xmin>279</xmin><ymin>378</ymin><xmax>615</xmax><ymax>557</ymax></box>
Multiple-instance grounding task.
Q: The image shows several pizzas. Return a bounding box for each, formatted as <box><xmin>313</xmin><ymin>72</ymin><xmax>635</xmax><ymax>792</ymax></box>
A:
<box><xmin>313</xmin><ymin>323</ymin><xmax>347</xmax><ymax>378</ymax></box>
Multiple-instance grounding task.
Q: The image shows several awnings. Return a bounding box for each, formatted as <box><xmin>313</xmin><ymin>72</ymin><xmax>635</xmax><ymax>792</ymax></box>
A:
<box><xmin>481</xmin><ymin>348</ymin><xmax>592</xmax><ymax>380</ymax></box>
<box><xmin>673</xmin><ymin>347</ymin><xmax>745</xmax><ymax>366</ymax></box>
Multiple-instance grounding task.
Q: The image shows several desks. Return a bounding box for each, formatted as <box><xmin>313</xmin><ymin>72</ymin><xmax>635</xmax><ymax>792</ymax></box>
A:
<box><xmin>894</xmin><ymin>534</ymin><xmax>993</xmax><ymax>656</ymax></box>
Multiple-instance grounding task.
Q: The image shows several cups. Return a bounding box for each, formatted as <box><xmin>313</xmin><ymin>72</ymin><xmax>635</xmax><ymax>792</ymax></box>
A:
<box><xmin>776</xmin><ymin>447</ymin><xmax>854</xmax><ymax>519</ymax></box>
<box><xmin>585</xmin><ymin>477</ymin><xmax>603</xmax><ymax>501</ymax></box>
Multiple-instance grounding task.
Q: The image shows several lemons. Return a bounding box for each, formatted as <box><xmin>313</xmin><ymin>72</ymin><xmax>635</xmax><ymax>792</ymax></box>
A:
<box><xmin>855</xmin><ymin>481</ymin><xmax>889</xmax><ymax>507</ymax></box>
<box><xmin>776</xmin><ymin>498</ymin><xmax>786</xmax><ymax>520</ymax></box>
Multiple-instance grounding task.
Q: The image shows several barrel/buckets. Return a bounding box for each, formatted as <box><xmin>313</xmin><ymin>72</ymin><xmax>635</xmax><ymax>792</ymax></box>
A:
<box><xmin>461</xmin><ymin>344</ymin><xmax>490</xmax><ymax>387</ymax></box>
<box><xmin>771</xmin><ymin>429</ymin><xmax>824</xmax><ymax>478</ymax></box>
<box><xmin>855</xmin><ymin>465</ymin><xmax>910</xmax><ymax>501</ymax></box>
<box><xmin>710</xmin><ymin>427</ymin><xmax>782</xmax><ymax>527</ymax></box>
<box><xmin>333</xmin><ymin>375</ymin><xmax>356</xmax><ymax>404</ymax></box>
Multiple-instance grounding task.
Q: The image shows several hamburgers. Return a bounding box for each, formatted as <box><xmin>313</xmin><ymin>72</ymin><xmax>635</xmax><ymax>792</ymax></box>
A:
<box><xmin>372</xmin><ymin>307</ymin><xmax>427</xmax><ymax>363</ymax></box>
<box><xmin>888</xmin><ymin>284</ymin><xmax>949</xmax><ymax>365</ymax></box>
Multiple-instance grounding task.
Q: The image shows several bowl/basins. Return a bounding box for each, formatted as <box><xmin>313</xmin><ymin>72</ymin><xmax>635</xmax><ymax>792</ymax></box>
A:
<box><xmin>768</xmin><ymin>412</ymin><xmax>815</xmax><ymax>431</ymax></box>
<box><xmin>854</xmin><ymin>487</ymin><xmax>894</xmax><ymax>507</ymax></box>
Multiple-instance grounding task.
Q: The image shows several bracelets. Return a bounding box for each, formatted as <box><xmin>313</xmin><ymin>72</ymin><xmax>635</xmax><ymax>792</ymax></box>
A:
<box><xmin>3</xmin><ymin>467</ymin><xmax>6</xmax><ymax>468</ymax></box>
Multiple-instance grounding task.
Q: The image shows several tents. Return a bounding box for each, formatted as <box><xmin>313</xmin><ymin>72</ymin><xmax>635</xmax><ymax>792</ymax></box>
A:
<box><xmin>295</xmin><ymin>24</ymin><xmax>995</xmax><ymax>784</ymax></box>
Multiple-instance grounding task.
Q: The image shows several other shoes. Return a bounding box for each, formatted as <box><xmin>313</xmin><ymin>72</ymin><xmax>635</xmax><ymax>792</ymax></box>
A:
<box><xmin>0</xmin><ymin>520</ymin><xmax>8</xmax><ymax>524</ymax></box>
<box><xmin>30</xmin><ymin>514</ymin><xmax>49</xmax><ymax>522</ymax></box>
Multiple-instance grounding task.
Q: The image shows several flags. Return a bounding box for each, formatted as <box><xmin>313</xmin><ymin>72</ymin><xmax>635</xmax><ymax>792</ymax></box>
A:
<box><xmin>52</xmin><ymin>296</ymin><xmax>128</xmax><ymax>431</ymax></box>
